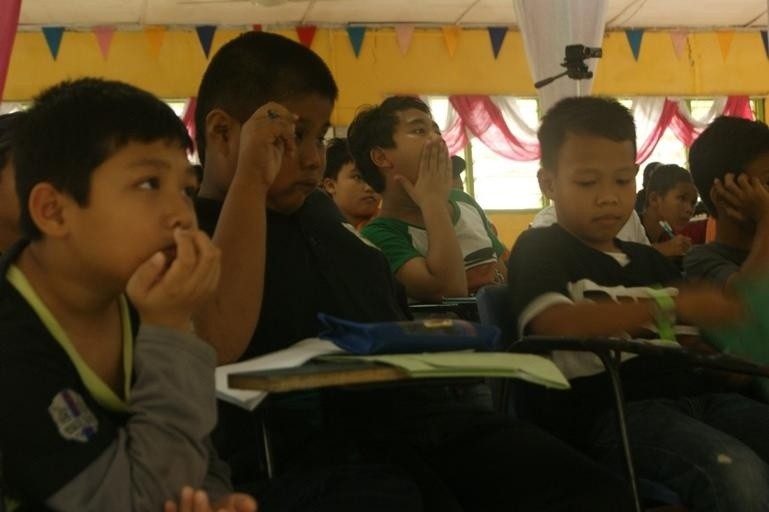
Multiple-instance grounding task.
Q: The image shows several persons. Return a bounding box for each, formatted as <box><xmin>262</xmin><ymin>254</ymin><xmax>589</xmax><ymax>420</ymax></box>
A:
<box><xmin>508</xmin><ymin>96</ymin><xmax>766</xmax><ymax>510</ymax></box>
<box><xmin>346</xmin><ymin>95</ymin><xmax>507</xmax><ymax>300</ymax></box>
<box><xmin>634</xmin><ymin>162</ymin><xmax>697</xmax><ymax>257</ymax></box>
<box><xmin>322</xmin><ymin>136</ymin><xmax>381</xmax><ymax>228</ymax></box>
<box><xmin>685</xmin><ymin>116</ymin><xmax>767</xmax><ymax>314</ymax></box>
<box><xmin>1</xmin><ymin>77</ymin><xmax>253</xmax><ymax>510</ymax></box>
<box><xmin>2</xmin><ymin>109</ymin><xmax>30</xmax><ymax>268</ymax></box>
<box><xmin>192</xmin><ymin>32</ymin><xmax>560</xmax><ymax>510</ymax></box>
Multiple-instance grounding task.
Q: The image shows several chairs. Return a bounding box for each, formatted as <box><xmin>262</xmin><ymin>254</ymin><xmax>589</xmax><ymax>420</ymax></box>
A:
<box><xmin>406</xmin><ymin>299</ymin><xmax>468</xmax><ymax>316</ymax></box>
<box><xmin>476</xmin><ymin>283</ymin><xmax>769</xmax><ymax>512</ymax></box>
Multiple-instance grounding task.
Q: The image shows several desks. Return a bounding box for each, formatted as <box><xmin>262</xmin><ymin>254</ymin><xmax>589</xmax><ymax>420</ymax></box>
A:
<box><xmin>226</xmin><ymin>348</ymin><xmax>561</xmax><ymax>488</ymax></box>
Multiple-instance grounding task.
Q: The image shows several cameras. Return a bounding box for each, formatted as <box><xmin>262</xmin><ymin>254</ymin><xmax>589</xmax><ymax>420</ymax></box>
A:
<box><xmin>560</xmin><ymin>44</ymin><xmax>602</xmax><ymax>80</ymax></box>
